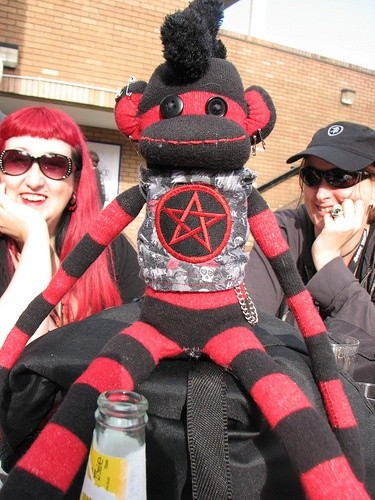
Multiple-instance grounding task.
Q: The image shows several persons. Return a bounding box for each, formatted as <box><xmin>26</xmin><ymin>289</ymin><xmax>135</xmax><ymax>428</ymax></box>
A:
<box><xmin>0</xmin><ymin>106</ymin><xmax>146</xmax><ymax>347</ymax></box>
<box><xmin>88</xmin><ymin>150</ymin><xmax>105</xmax><ymax>206</ymax></box>
<box><xmin>244</xmin><ymin>121</ymin><xmax>375</xmax><ymax>361</ymax></box>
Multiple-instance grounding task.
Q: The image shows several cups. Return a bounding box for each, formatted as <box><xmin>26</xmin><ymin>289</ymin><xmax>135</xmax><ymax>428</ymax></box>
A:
<box><xmin>327</xmin><ymin>332</ymin><xmax>360</xmax><ymax>379</ymax></box>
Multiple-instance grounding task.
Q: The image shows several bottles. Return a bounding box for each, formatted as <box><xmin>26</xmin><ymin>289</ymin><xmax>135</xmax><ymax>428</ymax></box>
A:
<box><xmin>80</xmin><ymin>388</ymin><xmax>150</xmax><ymax>500</ymax></box>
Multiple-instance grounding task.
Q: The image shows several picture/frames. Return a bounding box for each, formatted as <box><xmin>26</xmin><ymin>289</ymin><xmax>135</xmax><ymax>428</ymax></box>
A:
<box><xmin>87</xmin><ymin>140</ymin><xmax>122</xmax><ymax>208</ymax></box>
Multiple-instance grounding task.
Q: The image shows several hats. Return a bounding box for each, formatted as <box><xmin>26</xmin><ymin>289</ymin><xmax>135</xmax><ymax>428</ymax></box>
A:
<box><xmin>286</xmin><ymin>121</ymin><xmax>375</xmax><ymax>173</ymax></box>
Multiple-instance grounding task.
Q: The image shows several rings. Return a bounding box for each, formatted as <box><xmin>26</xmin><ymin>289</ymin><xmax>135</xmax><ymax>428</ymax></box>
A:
<box><xmin>332</xmin><ymin>208</ymin><xmax>343</xmax><ymax>217</ymax></box>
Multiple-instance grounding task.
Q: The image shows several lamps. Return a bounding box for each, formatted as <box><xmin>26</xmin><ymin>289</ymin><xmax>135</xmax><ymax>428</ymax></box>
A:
<box><xmin>0</xmin><ymin>42</ymin><xmax>19</xmax><ymax>68</ymax></box>
<box><xmin>341</xmin><ymin>88</ymin><xmax>356</xmax><ymax>105</ymax></box>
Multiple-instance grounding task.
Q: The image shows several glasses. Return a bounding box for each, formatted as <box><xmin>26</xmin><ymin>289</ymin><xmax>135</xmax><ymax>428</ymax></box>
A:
<box><xmin>0</xmin><ymin>148</ymin><xmax>80</xmax><ymax>182</ymax></box>
<box><xmin>299</xmin><ymin>166</ymin><xmax>375</xmax><ymax>190</ymax></box>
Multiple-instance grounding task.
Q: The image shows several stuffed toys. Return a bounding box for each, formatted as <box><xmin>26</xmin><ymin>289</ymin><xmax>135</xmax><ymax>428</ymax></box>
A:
<box><xmin>0</xmin><ymin>0</ymin><xmax>373</xmax><ymax>500</ymax></box>
